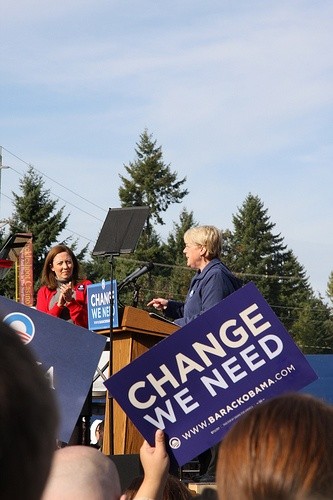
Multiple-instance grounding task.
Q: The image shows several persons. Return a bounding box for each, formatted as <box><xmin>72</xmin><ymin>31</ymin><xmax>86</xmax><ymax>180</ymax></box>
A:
<box><xmin>133</xmin><ymin>430</ymin><xmax>170</xmax><ymax>500</ymax></box>
<box><xmin>119</xmin><ymin>472</ymin><xmax>194</xmax><ymax>500</ymax></box>
<box><xmin>0</xmin><ymin>320</ymin><xmax>121</xmax><ymax>500</ymax></box>
<box><xmin>36</xmin><ymin>245</ymin><xmax>100</xmax><ymax>448</ymax></box>
<box><xmin>147</xmin><ymin>226</ymin><xmax>244</xmax><ymax>483</ymax></box>
<box><xmin>40</xmin><ymin>445</ymin><xmax>121</xmax><ymax>500</ymax></box>
<box><xmin>215</xmin><ymin>393</ymin><xmax>333</xmax><ymax>500</ymax></box>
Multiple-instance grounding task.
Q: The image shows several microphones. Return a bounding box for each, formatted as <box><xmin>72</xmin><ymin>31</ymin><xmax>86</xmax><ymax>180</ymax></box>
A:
<box><xmin>116</xmin><ymin>268</ymin><xmax>141</xmax><ymax>309</ymax></box>
<box><xmin>118</xmin><ymin>266</ymin><xmax>148</xmax><ymax>307</ymax></box>
